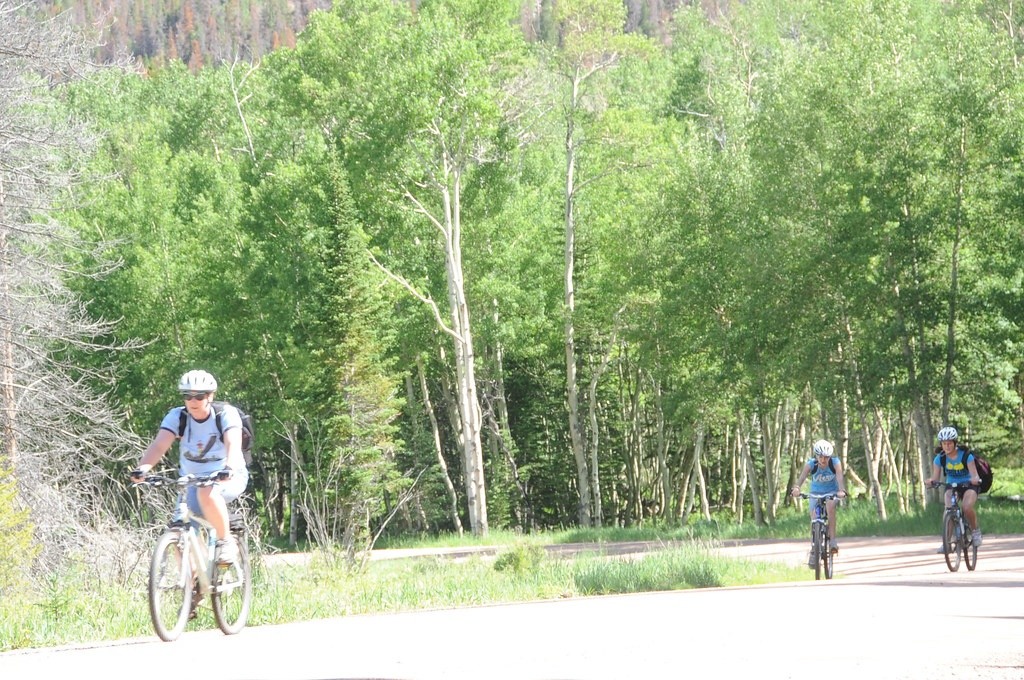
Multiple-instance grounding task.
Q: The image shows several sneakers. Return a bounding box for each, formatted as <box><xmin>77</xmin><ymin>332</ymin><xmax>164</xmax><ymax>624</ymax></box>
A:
<box><xmin>215</xmin><ymin>540</ymin><xmax>239</xmax><ymax>565</ymax></box>
<box><xmin>178</xmin><ymin>593</ymin><xmax>205</xmax><ymax>621</ymax></box>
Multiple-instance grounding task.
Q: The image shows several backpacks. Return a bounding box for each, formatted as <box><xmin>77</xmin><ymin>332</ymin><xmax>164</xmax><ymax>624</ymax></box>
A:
<box><xmin>940</xmin><ymin>445</ymin><xmax>992</xmax><ymax>494</ymax></box>
<box><xmin>178</xmin><ymin>402</ymin><xmax>253</xmax><ymax>451</ymax></box>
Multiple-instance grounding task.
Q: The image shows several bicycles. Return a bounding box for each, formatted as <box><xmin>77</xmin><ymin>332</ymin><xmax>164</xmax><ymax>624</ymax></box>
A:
<box><xmin>789</xmin><ymin>488</ymin><xmax>849</xmax><ymax>580</ymax></box>
<box><xmin>923</xmin><ymin>479</ymin><xmax>983</xmax><ymax>573</ymax></box>
<box><xmin>127</xmin><ymin>470</ymin><xmax>253</xmax><ymax>642</ymax></box>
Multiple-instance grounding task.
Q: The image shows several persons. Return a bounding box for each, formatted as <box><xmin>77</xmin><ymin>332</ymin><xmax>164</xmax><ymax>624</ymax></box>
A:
<box><xmin>925</xmin><ymin>426</ymin><xmax>983</xmax><ymax>554</ymax></box>
<box><xmin>791</xmin><ymin>440</ymin><xmax>845</xmax><ymax>570</ymax></box>
<box><xmin>131</xmin><ymin>370</ymin><xmax>249</xmax><ymax>621</ymax></box>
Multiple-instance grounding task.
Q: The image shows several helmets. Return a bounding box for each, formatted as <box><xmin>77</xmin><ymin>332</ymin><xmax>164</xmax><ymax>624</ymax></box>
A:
<box><xmin>814</xmin><ymin>440</ymin><xmax>833</xmax><ymax>457</ymax></box>
<box><xmin>178</xmin><ymin>369</ymin><xmax>218</xmax><ymax>396</ymax></box>
<box><xmin>937</xmin><ymin>427</ymin><xmax>958</xmax><ymax>443</ymax></box>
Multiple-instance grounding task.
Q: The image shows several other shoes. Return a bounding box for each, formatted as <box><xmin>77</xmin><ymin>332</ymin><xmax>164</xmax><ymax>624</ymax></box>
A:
<box><xmin>829</xmin><ymin>541</ymin><xmax>838</xmax><ymax>552</ymax></box>
<box><xmin>937</xmin><ymin>545</ymin><xmax>951</xmax><ymax>553</ymax></box>
<box><xmin>809</xmin><ymin>550</ymin><xmax>817</xmax><ymax>567</ymax></box>
<box><xmin>972</xmin><ymin>528</ymin><xmax>981</xmax><ymax>546</ymax></box>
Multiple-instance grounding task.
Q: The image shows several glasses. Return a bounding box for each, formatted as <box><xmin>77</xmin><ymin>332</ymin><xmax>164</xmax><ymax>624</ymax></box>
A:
<box><xmin>182</xmin><ymin>392</ymin><xmax>211</xmax><ymax>401</ymax></box>
